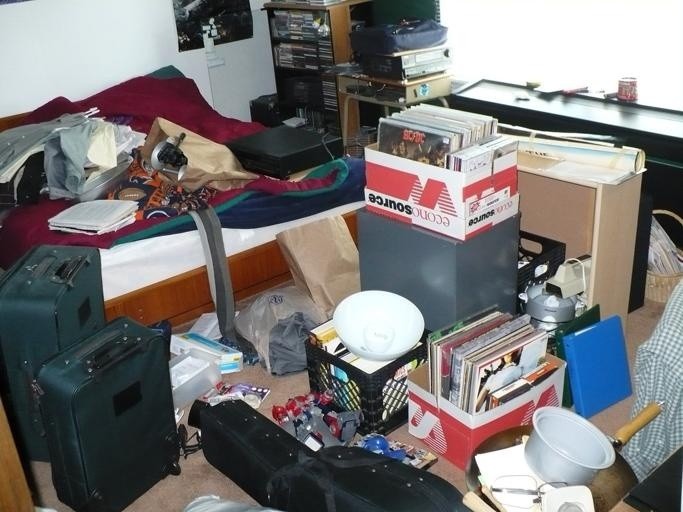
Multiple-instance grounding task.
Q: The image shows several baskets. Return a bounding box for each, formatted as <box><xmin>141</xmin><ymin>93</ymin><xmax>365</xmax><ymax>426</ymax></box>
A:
<box><xmin>516</xmin><ymin>231</ymin><xmax>565</xmax><ymax>316</ymax></box>
<box><xmin>643</xmin><ymin>209</ymin><xmax>682</xmax><ymax>303</ymax></box>
<box><xmin>304</xmin><ymin>328</ymin><xmax>436</xmax><ymax>435</ymax></box>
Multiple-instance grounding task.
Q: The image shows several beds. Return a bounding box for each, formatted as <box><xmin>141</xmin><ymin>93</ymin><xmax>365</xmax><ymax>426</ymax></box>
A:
<box><xmin>2</xmin><ymin>63</ymin><xmax>368</xmax><ymax>330</ymax></box>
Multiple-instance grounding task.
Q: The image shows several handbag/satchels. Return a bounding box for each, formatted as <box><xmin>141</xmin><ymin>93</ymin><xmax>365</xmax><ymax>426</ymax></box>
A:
<box><xmin>385</xmin><ymin>17</ymin><xmax>447</xmax><ymax>52</ymax></box>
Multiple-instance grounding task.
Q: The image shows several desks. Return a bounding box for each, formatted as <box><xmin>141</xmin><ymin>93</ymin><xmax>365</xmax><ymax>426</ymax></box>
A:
<box><xmin>337</xmin><ymin>71</ymin><xmax>452</xmax><ymax>150</ymax></box>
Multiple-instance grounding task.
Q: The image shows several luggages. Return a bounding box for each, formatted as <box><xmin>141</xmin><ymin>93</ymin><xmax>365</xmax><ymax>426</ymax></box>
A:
<box><xmin>1</xmin><ymin>245</ymin><xmax>107</xmax><ymax>507</ymax></box>
<box><xmin>31</xmin><ymin>316</ymin><xmax>181</xmax><ymax>511</ymax></box>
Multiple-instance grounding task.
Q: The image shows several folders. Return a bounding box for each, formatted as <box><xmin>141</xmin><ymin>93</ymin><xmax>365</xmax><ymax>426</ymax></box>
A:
<box><xmin>555</xmin><ymin>304</ymin><xmax>632</xmax><ymax>421</ymax></box>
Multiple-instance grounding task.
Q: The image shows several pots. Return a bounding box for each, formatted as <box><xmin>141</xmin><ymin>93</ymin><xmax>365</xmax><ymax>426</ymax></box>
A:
<box><xmin>465</xmin><ymin>398</ymin><xmax>664</xmax><ymax>510</ymax></box>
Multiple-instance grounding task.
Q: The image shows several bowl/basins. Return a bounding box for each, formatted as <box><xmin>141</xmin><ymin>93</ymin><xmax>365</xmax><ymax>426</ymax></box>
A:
<box><xmin>331</xmin><ymin>287</ymin><xmax>423</xmax><ymax>362</ymax></box>
<box><xmin>524</xmin><ymin>404</ymin><xmax>615</xmax><ymax>486</ymax></box>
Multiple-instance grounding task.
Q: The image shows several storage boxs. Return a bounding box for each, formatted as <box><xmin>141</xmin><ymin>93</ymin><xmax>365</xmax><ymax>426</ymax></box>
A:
<box><xmin>405</xmin><ymin>354</ymin><xmax>568</xmax><ymax>470</ymax></box>
<box><xmin>356</xmin><ymin>208</ymin><xmax>519</xmax><ymax>333</ymax></box>
<box><xmin>519</xmin><ymin>165</ymin><xmax>656</xmax><ymax>337</ymax></box>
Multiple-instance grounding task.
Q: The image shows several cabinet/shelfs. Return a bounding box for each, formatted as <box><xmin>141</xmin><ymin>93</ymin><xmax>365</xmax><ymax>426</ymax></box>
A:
<box><xmin>262</xmin><ymin>2</ymin><xmax>364</xmax><ymax>158</ymax></box>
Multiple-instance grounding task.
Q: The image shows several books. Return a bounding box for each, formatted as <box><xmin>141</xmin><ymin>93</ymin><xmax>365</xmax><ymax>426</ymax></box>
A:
<box><xmin>429</xmin><ymin>302</ymin><xmax>560</xmax><ymax>418</ymax></box>
<box><xmin>47</xmin><ymin>197</ymin><xmax>139</xmax><ymax>235</ymax></box>
<box><xmin>378</xmin><ymin>102</ymin><xmax>521</xmax><ymax>173</ymax></box>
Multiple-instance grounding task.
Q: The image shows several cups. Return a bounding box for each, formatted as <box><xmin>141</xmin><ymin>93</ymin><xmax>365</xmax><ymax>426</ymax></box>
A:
<box><xmin>616</xmin><ymin>76</ymin><xmax>639</xmax><ymax>101</ymax></box>
